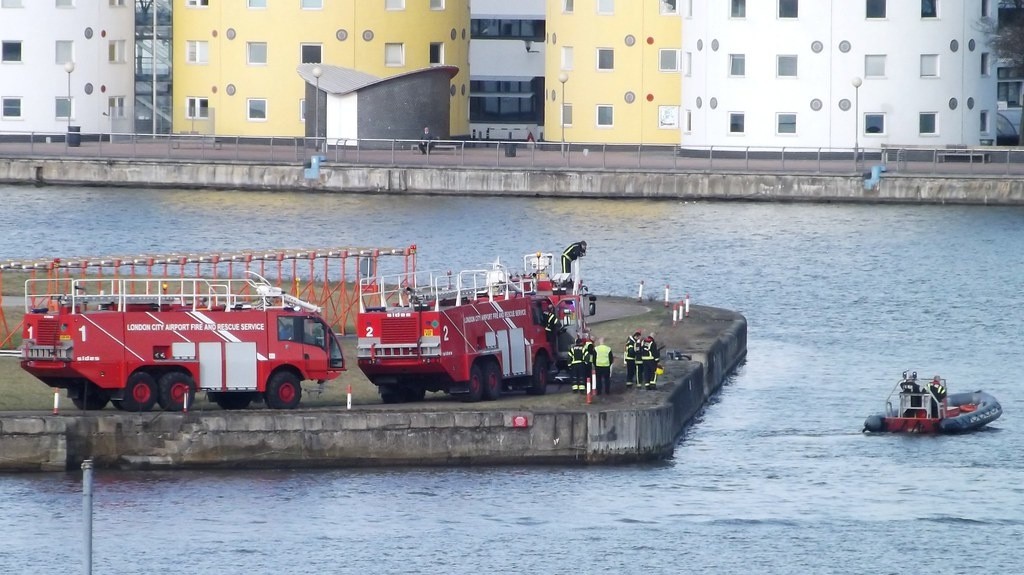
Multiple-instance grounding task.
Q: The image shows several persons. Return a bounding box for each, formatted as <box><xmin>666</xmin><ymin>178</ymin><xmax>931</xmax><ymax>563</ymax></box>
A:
<box><xmin>561</xmin><ymin>241</ymin><xmax>587</xmax><ymax>273</ymax></box>
<box><xmin>279</xmin><ymin>324</ymin><xmax>292</xmax><ymax>340</ymax></box>
<box><xmin>922</xmin><ymin>376</ymin><xmax>946</xmax><ymax>418</ymax></box>
<box><xmin>419</xmin><ymin>127</ymin><xmax>434</xmax><ymax>155</ymax></box>
<box><xmin>567</xmin><ymin>337</ymin><xmax>614</xmax><ymax>395</ymax></box>
<box><xmin>624</xmin><ymin>332</ymin><xmax>660</xmax><ymax>389</ymax></box>
<box><xmin>107</xmin><ymin>302</ymin><xmax>116</xmax><ymax>312</ymax></box>
<box><xmin>543</xmin><ymin>304</ymin><xmax>559</xmax><ymax>342</ymax></box>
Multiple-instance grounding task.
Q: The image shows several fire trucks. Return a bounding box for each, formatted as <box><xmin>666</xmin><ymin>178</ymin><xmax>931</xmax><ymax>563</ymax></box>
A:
<box><xmin>356</xmin><ymin>252</ymin><xmax>596</xmax><ymax>404</ymax></box>
<box><xmin>20</xmin><ymin>270</ymin><xmax>348</xmax><ymax>411</ymax></box>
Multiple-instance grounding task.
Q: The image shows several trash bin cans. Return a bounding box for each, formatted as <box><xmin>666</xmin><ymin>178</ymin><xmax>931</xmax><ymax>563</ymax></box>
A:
<box><xmin>980</xmin><ymin>139</ymin><xmax>994</xmax><ymax>146</ymax></box>
<box><xmin>68</xmin><ymin>126</ymin><xmax>81</xmax><ymax>146</ymax></box>
<box><xmin>505</xmin><ymin>139</ymin><xmax>516</xmax><ymax>157</ymax></box>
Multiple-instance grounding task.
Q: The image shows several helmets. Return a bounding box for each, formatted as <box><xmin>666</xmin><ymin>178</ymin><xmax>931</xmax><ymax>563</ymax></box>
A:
<box><xmin>656</xmin><ymin>367</ymin><xmax>665</xmax><ymax>374</ymax></box>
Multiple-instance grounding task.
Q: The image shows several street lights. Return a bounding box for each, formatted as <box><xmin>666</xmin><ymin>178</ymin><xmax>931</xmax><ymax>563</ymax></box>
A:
<box><xmin>312</xmin><ymin>66</ymin><xmax>322</xmax><ymax>150</ymax></box>
<box><xmin>65</xmin><ymin>62</ymin><xmax>74</xmax><ymax>131</ymax></box>
<box><xmin>558</xmin><ymin>72</ymin><xmax>568</xmax><ymax>158</ymax></box>
<box><xmin>852</xmin><ymin>77</ymin><xmax>863</xmax><ymax>150</ymax></box>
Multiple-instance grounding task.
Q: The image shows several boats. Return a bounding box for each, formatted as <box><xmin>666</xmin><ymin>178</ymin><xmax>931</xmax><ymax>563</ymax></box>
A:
<box><xmin>862</xmin><ymin>372</ymin><xmax>1003</xmax><ymax>434</ymax></box>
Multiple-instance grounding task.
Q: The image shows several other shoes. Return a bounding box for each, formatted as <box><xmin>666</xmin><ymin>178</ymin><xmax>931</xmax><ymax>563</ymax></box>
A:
<box><xmin>580</xmin><ymin>390</ymin><xmax>584</xmax><ymax>393</ymax></box>
<box><xmin>645</xmin><ymin>386</ymin><xmax>651</xmax><ymax>390</ymax></box>
<box><xmin>650</xmin><ymin>385</ymin><xmax>658</xmax><ymax>390</ymax></box>
<box><xmin>605</xmin><ymin>390</ymin><xmax>609</xmax><ymax>394</ymax></box>
<box><xmin>573</xmin><ymin>389</ymin><xmax>577</xmax><ymax>393</ymax></box>
<box><xmin>598</xmin><ymin>390</ymin><xmax>602</xmax><ymax>394</ymax></box>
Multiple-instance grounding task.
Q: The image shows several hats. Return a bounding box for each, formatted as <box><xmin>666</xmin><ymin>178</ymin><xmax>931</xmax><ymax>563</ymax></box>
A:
<box><xmin>590</xmin><ymin>336</ymin><xmax>596</xmax><ymax>342</ymax></box>
<box><xmin>648</xmin><ymin>332</ymin><xmax>657</xmax><ymax>340</ymax></box>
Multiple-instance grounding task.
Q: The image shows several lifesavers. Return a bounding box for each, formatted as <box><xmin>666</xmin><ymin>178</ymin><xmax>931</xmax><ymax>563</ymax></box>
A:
<box><xmin>959</xmin><ymin>404</ymin><xmax>977</xmax><ymax>412</ymax></box>
